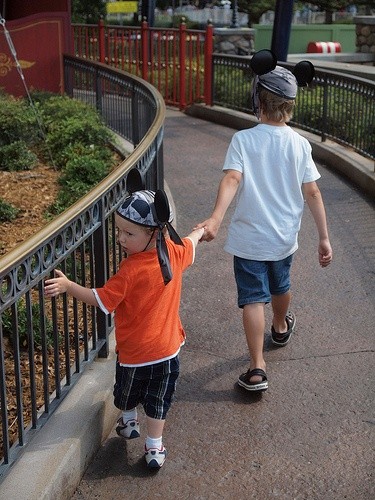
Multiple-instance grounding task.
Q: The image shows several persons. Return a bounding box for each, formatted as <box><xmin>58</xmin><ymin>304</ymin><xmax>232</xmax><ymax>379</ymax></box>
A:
<box><xmin>193</xmin><ymin>49</ymin><xmax>332</xmax><ymax>393</ymax></box>
<box><xmin>44</xmin><ymin>168</ymin><xmax>204</xmax><ymax>468</ymax></box>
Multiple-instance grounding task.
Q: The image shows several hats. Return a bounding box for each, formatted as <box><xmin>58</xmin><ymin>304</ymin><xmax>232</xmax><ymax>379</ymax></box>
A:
<box><xmin>252</xmin><ymin>49</ymin><xmax>315</xmax><ymax>100</ymax></box>
<box><xmin>117</xmin><ymin>165</ymin><xmax>175</xmax><ymax>227</ymax></box>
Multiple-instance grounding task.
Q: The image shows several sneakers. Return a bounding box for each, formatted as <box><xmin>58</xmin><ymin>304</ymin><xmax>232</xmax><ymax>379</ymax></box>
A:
<box><xmin>142</xmin><ymin>442</ymin><xmax>168</xmax><ymax>468</ymax></box>
<box><xmin>115</xmin><ymin>417</ymin><xmax>142</xmax><ymax>439</ymax></box>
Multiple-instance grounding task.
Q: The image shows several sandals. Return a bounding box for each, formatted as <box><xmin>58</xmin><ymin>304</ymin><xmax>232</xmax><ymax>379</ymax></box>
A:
<box><xmin>271</xmin><ymin>310</ymin><xmax>296</xmax><ymax>346</ymax></box>
<box><xmin>237</xmin><ymin>367</ymin><xmax>271</xmax><ymax>392</ymax></box>
